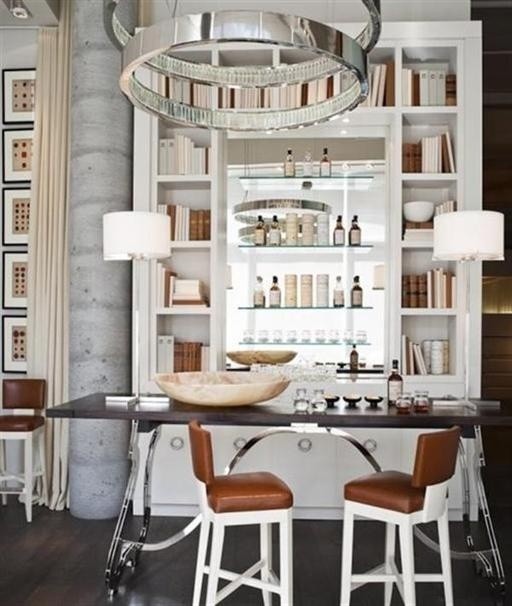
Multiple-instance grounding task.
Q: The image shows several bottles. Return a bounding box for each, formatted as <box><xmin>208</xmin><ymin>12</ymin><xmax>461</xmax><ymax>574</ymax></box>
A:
<box><xmin>348</xmin><ymin>215</ymin><xmax>361</xmax><ymax>246</ymax></box>
<box><xmin>387</xmin><ymin>359</ymin><xmax>403</xmax><ymax>406</ymax></box>
<box><xmin>285</xmin><ymin>273</ymin><xmax>297</xmax><ymax>308</ymax></box>
<box><xmin>320</xmin><ymin>148</ymin><xmax>331</xmax><ymax>178</ymax></box>
<box><xmin>286</xmin><ymin>212</ymin><xmax>297</xmax><ymax>246</ymax></box>
<box><xmin>285</xmin><ymin>147</ymin><xmax>295</xmax><ymax>177</ymax></box>
<box><xmin>254</xmin><ymin>216</ymin><xmax>266</xmax><ymax>247</ymax></box>
<box><xmin>350</xmin><ymin>344</ymin><xmax>359</xmax><ymax>371</ymax></box>
<box><xmin>316</xmin><ymin>213</ymin><xmax>329</xmax><ymax>246</ymax></box>
<box><xmin>333</xmin><ymin>277</ymin><xmax>344</xmax><ymax>308</ymax></box>
<box><xmin>269</xmin><ymin>276</ymin><xmax>280</xmax><ymax>308</ymax></box>
<box><xmin>301</xmin><ymin>275</ymin><xmax>313</xmax><ymax>309</ymax></box>
<box><xmin>316</xmin><ymin>274</ymin><xmax>329</xmax><ymax>307</ymax></box>
<box><xmin>253</xmin><ymin>275</ymin><xmax>266</xmax><ymax>307</ymax></box>
<box><xmin>351</xmin><ymin>276</ymin><xmax>363</xmax><ymax>307</ymax></box>
<box><xmin>334</xmin><ymin>215</ymin><xmax>345</xmax><ymax>246</ymax></box>
<box><xmin>269</xmin><ymin>216</ymin><xmax>280</xmax><ymax>246</ymax></box>
<box><xmin>302</xmin><ymin>149</ymin><xmax>312</xmax><ymax>177</ymax></box>
<box><xmin>302</xmin><ymin>214</ymin><xmax>316</xmax><ymax>247</ymax></box>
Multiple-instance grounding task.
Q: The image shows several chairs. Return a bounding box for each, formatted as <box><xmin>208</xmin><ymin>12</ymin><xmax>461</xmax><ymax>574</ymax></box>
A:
<box><xmin>340</xmin><ymin>425</ymin><xmax>461</xmax><ymax>606</ymax></box>
<box><xmin>189</xmin><ymin>419</ymin><xmax>293</xmax><ymax>606</ymax></box>
<box><xmin>0</xmin><ymin>379</ymin><xmax>46</xmax><ymax>522</ymax></box>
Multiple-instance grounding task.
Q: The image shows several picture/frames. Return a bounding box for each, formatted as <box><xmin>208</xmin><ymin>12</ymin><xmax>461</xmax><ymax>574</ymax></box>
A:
<box><xmin>2</xmin><ymin>251</ymin><xmax>27</xmax><ymax>310</ymax></box>
<box><xmin>1</xmin><ymin>67</ymin><xmax>36</xmax><ymax>124</ymax></box>
<box><xmin>2</xmin><ymin>127</ymin><xmax>34</xmax><ymax>183</ymax></box>
<box><xmin>2</xmin><ymin>187</ymin><xmax>31</xmax><ymax>246</ymax></box>
<box><xmin>1</xmin><ymin>315</ymin><xmax>27</xmax><ymax>374</ymax></box>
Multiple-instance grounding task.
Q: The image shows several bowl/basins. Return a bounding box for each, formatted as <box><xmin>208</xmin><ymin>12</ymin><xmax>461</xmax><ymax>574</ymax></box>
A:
<box><xmin>155</xmin><ymin>372</ymin><xmax>290</xmax><ymax>407</ymax></box>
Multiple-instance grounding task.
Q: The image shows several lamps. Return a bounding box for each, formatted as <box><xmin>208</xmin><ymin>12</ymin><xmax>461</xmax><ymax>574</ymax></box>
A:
<box><xmin>102</xmin><ymin>211</ymin><xmax>171</xmax><ymax>406</ymax></box>
<box><xmin>433</xmin><ymin>211</ymin><xmax>504</xmax><ymax>409</ymax></box>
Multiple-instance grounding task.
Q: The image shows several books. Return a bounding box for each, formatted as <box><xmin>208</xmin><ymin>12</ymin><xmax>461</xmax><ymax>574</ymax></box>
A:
<box><xmin>433</xmin><ymin>198</ymin><xmax>455</xmax><ymax>219</ymax></box>
<box><xmin>367</xmin><ymin>56</ymin><xmax>396</xmax><ymax>107</ymax></box>
<box><xmin>158</xmin><ymin>135</ymin><xmax>212</xmax><ymax>175</ymax></box>
<box><xmin>401</xmin><ymin>66</ymin><xmax>447</xmax><ymax>106</ymax></box>
<box><xmin>156</xmin><ymin>263</ymin><xmax>207</xmax><ymax>308</ymax></box>
<box><xmin>400</xmin><ymin>265</ymin><xmax>455</xmax><ymax>308</ymax></box>
<box><xmin>157</xmin><ymin>76</ymin><xmax>345</xmax><ymax>109</ymax></box>
<box><xmin>156</xmin><ymin>202</ymin><xmax>210</xmax><ymax>241</ymax></box>
<box><xmin>158</xmin><ymin>335</ymin><xmax>208</xmax><ymax>372</ymax></box>
<box><xmin>404</xmin><ymin>131</ymin><xmax>455</xmax><ymax>173</ymax></box>
<box><xmin>400</xmin><ymin>333</ymin><xmax>426</xmax><ymax>375</ymax></box>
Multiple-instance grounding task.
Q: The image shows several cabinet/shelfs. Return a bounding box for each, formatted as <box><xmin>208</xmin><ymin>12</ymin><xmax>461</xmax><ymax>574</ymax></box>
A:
<box><xmin>132</xmin><ymin>19</ymin><xmax>484</xmax><ymax>522</ymax></box>
